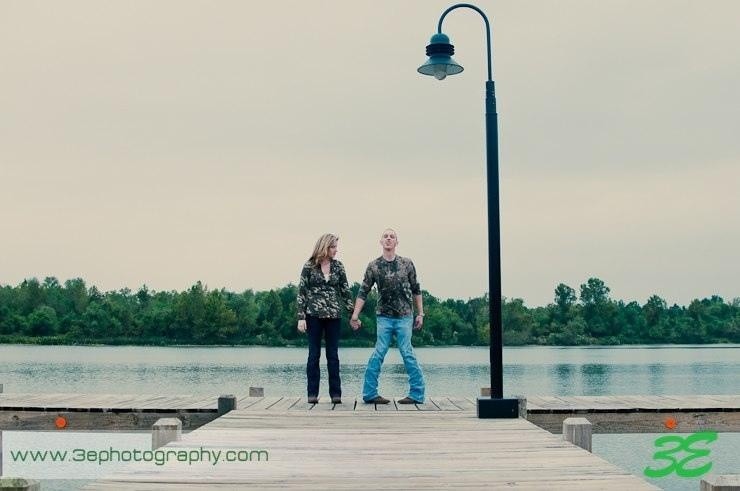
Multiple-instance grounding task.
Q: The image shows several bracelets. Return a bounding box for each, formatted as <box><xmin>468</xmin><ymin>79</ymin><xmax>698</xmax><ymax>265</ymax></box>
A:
<box><xmin>417</xmin><ymin>313</ymin><xmax>426</xmax><ymax>318</ymax></box>
<box><xmin>350</xmin><ymin>318</ymin><xmax>358</xmax><ymax>322</ymax></box>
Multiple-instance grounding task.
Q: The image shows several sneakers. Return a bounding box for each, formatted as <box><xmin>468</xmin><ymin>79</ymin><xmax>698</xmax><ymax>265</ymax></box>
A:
<box><xmin>364</xmin><ymin>395</ymin><xmax>390</xmax><ymax>404</ymax></box>
<box><xmin>330</xmin><ymin>396</ymin><xmax>342</xmax><ymax>404</ymax></box>
<box><xmin>398</xmin><ymin>397</ymin><xmax>422</xmax><ymax>405</ymax></box>
<box><xmin>307</xmin><ymin>396</ymin><xmax>319</xmax><ymax>404</ymax></box>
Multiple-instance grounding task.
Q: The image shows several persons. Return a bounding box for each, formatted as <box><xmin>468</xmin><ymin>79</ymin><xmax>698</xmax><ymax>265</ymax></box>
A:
<box><xmin>293</xmin><ymin>232</ymin><xmax>353</xmax><ymax>404</ymax></box>
<box><xmin>347</xmin><ymin>228</ymin><xmax>428</xmax><ymax>406</ymax></box>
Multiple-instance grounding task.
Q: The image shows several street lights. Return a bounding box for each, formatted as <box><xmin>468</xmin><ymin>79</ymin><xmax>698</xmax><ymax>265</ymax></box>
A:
<box><xmin>417</xmin><ymin>4</ymin><xmax>520</xmax><ymax>421</ymax></box>
